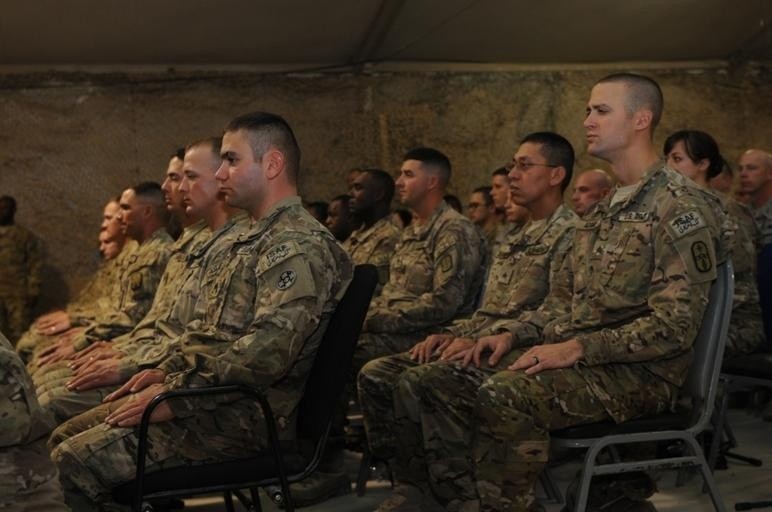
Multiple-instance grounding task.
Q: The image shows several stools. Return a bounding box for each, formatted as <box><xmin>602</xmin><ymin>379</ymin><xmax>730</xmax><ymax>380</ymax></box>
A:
<box><xmin>697</xmin><ymin>366</ymin><xmax>769</xmax><ymax>494</ymax></box>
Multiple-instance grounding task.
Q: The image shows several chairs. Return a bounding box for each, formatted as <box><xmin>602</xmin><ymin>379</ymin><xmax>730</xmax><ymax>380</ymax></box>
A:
<box><xmin>540</xmin><ymin>256</ymin><xmax>737</xmax><ymax>510</ymax></box>
<box><xmin>113</xmin><ymin>261</ymin><xmax>380</xmax><ymax>507</ymax></box>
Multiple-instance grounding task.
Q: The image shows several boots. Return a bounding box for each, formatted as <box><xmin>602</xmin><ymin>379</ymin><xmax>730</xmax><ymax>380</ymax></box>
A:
<box><xmin>288</xmin><ymin>435</ymin><xmax>352</xmax><ymax>510</ymax></box>
<box><xmin>369</xmin><ymin>473</ymin><xmax>432</xmax><ymax>512</ymax></box>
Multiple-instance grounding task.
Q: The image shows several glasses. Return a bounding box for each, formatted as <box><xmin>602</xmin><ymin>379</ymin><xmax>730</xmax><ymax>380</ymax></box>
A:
<box><xmin>503</xmin><ymin>161</ymin><xmax>557</xmax><ymax>173</ymax></box>
